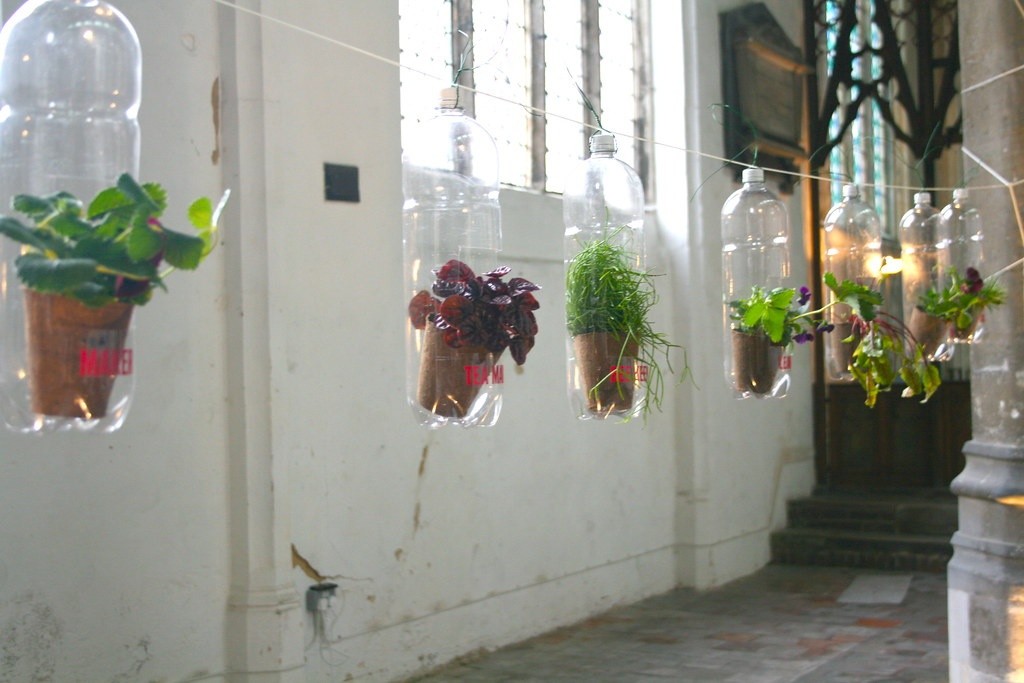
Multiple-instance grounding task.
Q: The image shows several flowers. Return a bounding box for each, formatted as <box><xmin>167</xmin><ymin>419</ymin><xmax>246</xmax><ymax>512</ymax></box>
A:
<box><xmin>725</xmin><ymin>271</ymin><xmax>887</xmax><ymax>345</ymax></box>
<box><xmin>917</xmin><ymin>264</ymin><xmax>985</xmax><ymax>327</ymax></box>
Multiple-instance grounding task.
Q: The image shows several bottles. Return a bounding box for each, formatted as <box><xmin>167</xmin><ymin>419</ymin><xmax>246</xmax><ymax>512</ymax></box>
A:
<box><xmin>824</xmin><ymin>185</ymin><xmax>883</xmax><ymax>379</ymax></box>
<box><xmin>941</xmin><ymin>189</ymin><xmax>985</xmax><ymax>345</ymax></box>
<box><xmin>564</xmin><ymin>134</ymin><xmax>648</xmax><ymax>421</ymax></box>
<box><xmin>720</xmin><ymin>168</ymin><xmax>791</xmax><ymax>399</ymax></box>
<box><xmin>402</xmin><ymin>88</ymin><xmax>501</xmax><ymax>431</ymax></box>
<box><xmin>0</xmin><ymin>0</ymin><xmax>141</xmax><ymax>436</ymax></box>
<box><xmin>898</xmin><ymin>193</ymin><xmax>953</xmax><ymax>363</ymax></box>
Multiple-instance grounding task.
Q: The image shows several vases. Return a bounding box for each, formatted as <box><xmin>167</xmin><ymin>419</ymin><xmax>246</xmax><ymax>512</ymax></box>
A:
<box><xmin>730</xmin><ymin>327</ymin><xmax>787</xmax><ymax>395</ymax></box>
<box><xmin>903</xmin><ymin>303</ymin><xmax>944</xmax><ymax>362</ymax></box>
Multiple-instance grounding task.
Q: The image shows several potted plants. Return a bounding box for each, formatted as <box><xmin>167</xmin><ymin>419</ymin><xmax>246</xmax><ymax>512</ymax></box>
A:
<box><xmin>946</xmin><ymin>275</ymin><xmax>1006</xmax><ymax>342</ymax></box>
<box><xmin>407</xmin><ymin>257</ymin><xmax>543</xmax><ymax>422</ymax></box>
<box><xmin>564</xmin><ymin>204</ymin><xmax>699</xmax><ymax>426</ymax></box>
<box><xmin>827</xmin><ymin>307</ymin><xmax>942</xmax><ymax>408</ymax></box>
<box><xmin>0</xmin><ymin>173</ymin><xmax>234</xmax><ymax>420</ymax></box>
